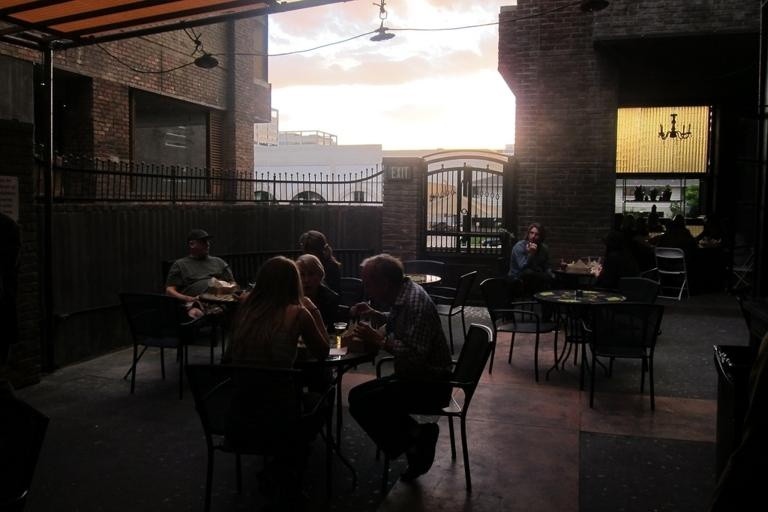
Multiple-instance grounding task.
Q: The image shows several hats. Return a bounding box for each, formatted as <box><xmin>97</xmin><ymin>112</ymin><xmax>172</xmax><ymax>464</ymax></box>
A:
<box><xmin>187</xmin><ymin>229</ymin><xmax>214</xmax><ymax>241</ymax></box>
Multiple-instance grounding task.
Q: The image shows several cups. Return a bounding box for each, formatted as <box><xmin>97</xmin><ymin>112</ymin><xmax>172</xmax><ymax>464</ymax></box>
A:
<box><xmin>560</xmin><ymin>262</ymin><xmax>567</xmax><ymax>271</ymax></box>
<box><xmin>207</xmin><ymin>279</ymin><xmax>215</xmax><ymax>294</ymax></box>
<box><xmin>334</xmin><ymin>322</ymin><xmax>348</xmax><ymax>336</ymax></box>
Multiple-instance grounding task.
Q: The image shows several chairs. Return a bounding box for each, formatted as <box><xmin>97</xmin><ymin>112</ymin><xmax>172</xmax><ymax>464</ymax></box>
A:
<box><xmin>637</xmin><ymin>247</ymin><xmax>689</xmax><ymax>304</ymax></box>
<box><xmin>724</xmin><ymin>248</ymin><xmax>757</xmax><ymax>295</ymax></box>
<box><xmin>574</xmin><ymin>300</ymin><xmax>663</xmax><ymax>414</ymax></box>
<box><xmin>329</xmin><ymin>277</ymin><xmax>369</xmax><ymax>333</ymax></box>
<box><xmin>181</xmin><ymin>360</ymin><xmax>332</xmax><ymax>512</ymax></box>
<box><xmin>373</xmin><ymin>324</ymin><xmax>495</xmax><ymax>497</ymax></box>
<box><xmin>119</xmin><ymin>293</ymin><xmax>221</xmax><ymax>394</ymax></box>
<box><xmin>618</xmin><ymin>275</ymin><xmax>662</xmax><ymax>304</ymax></box>
<box><xmin>478</xmin><ymin>270</ymin><xmax>560</xmax><ymax>384</ymax></box>
<box><xmin>396</xmin><ymin>256</ymin><xmax>481</xmax><ymax>356</ymax></box>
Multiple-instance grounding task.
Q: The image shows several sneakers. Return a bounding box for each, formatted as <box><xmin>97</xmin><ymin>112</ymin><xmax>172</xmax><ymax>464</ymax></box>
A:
<box><xmin>400</xmin><ymin>423</ymin><xmax>438</xmax><ymax>480</ymax></box>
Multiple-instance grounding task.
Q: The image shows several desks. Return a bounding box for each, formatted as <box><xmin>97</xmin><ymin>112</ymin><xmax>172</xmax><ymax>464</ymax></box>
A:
<box><xmin>285</xmin><ymin>329</ymin><xmax>382</xmax><ymax>492</ymax></box>
<box><xmin>534</xmin><ymin>280</ymin><xmax>628</xmax><ymax>392</ymax></box>
<box><xmin>195</xmin><ymin>291</ymin><xmax>247</xmax><ymax>305</ymax></box>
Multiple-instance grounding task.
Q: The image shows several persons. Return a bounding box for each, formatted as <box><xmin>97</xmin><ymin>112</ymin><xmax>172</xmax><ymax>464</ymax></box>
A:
<box><xmin>295</xmin><ymin>254</ymin><xmax>345</xmax><ymax>334</ymax></box>
<box><xmin>602</xmin><ymin>214</ymin><xmax>698</xmax><ymax>288</ymax></box>
<box><xmin>347</xmin><ymin>252</ymin><xmax>452</xmax><ymax>483</ymax></box>
<box><xmin>223</xmin><ymin>255</ymin><xmax>329</xmax><ymax>498</ymax></box>
<box><xmin>508</xmin><ymin>222</ymin><xmax>555</xmax><ymax>323</ymax></box>
<box><xmin>298</xmin><ymin>229</ymin><xmax>343</xmax><ymax>293</ymax></box>
<box><xmin>164</xmin><ymin>228</ymin><xmax>240</xmax><ymax>320</ymax></box>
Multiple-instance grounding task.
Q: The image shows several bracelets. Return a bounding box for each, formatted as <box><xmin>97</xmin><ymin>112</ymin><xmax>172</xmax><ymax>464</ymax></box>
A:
<box><xmin>310</xmin><ymin>307</ymin><xmax>318</xmax><ymax>314</ymax></box>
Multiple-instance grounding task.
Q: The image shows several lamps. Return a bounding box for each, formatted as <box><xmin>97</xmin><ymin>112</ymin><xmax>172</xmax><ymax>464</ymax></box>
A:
<box><xmin>194</xmin><ymin>48</ymin><xmax>218</xmax><ymax>69</ymax></box>
<box><xmin>370</xmin><ymin>21</ymin><xmax>395</xmax><ymax>40</ymax></box>
<box><xmin>657</xmin><ymin>113</ymin><xmax>693</xmax><ymax>141</ymax></box>
